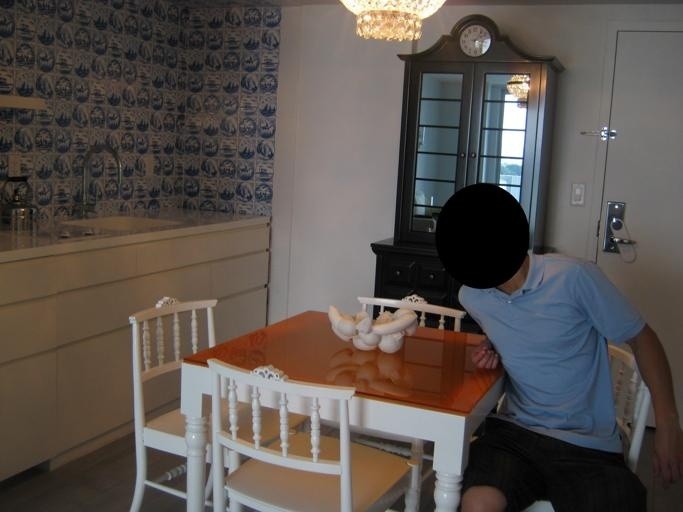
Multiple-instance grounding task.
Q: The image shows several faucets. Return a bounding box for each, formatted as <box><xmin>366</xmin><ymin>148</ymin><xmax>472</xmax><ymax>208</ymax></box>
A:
<box><xmin>79</xmin><ymin>142</ymin><xmax>122</xmax><ymax>218</ymax></box>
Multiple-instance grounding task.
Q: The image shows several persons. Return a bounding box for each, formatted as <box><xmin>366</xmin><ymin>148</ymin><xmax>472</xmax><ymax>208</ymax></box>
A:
<box><xmin>436</xmin><ymin>184</ymin><xmax>683</xmax><ymax>512</ymax></box>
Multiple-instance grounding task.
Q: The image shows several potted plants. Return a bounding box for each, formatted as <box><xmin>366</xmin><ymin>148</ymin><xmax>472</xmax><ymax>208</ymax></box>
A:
<box><xmin>341</xmin><ymin>0</ymin><xmax>446</xmax><ymax>41</ymax></box>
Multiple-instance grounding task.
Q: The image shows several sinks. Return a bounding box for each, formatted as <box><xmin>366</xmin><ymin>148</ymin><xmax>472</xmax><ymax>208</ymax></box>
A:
<box><xmin>62</xmin><ymin>215</ymin><xmax>185</xmax><ymax>233</ymax></box>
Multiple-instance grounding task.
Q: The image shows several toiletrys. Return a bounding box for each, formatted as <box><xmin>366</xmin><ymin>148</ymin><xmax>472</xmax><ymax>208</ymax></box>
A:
<box><xmin>10</xmin><ymin>185</ymin><xmax>31</xmax><ymax>230</ymax></box>
